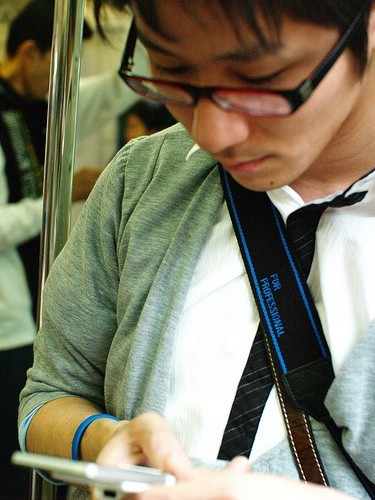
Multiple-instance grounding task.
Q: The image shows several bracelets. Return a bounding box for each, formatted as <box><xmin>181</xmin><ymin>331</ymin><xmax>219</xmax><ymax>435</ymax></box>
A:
<box><xmin>71</xmin><ymin>414</ymin><xmax>121</xmax><ymax>460</ymax></box>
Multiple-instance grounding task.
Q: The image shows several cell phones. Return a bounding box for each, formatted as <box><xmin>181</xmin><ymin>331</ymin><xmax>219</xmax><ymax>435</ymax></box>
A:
<box><xmin>12</xmin><ymin>451</ymin><xmax>178</xmax><ymax>494</ymax></box>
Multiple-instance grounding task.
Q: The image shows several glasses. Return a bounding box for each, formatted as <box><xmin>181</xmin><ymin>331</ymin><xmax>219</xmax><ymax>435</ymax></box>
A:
<box><xmin>117</xmin><ymin>15</ymin><xmax>366</xmax><ymax>120</ymax></box>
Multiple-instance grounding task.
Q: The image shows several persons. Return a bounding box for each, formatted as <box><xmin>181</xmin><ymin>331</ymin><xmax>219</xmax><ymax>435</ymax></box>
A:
<box><xmin>115</xmin><ymin>97</ymin><xmax>177</xmax><ymax>151</ymax></box>
<box><xmin>17</xmin><ymin>0</ymin><xmax>374</xmax><ymax>500</ymax></box>
<box><xmin>0</xmin><ymin>1</ymin><xmax>102</xmax><ymax>500</ymax></box>
